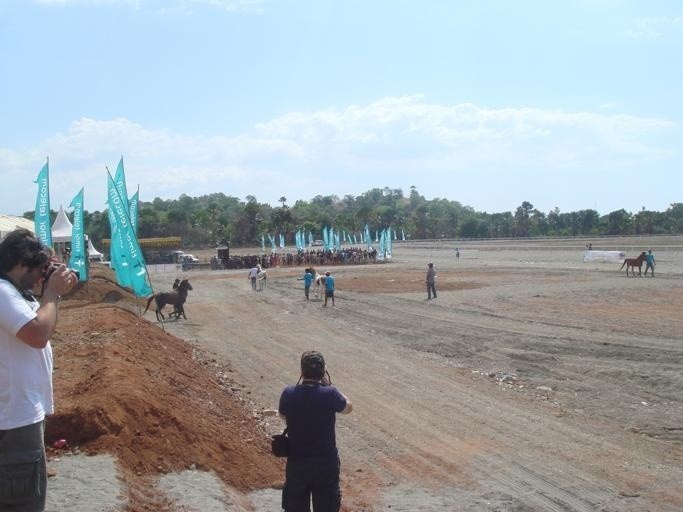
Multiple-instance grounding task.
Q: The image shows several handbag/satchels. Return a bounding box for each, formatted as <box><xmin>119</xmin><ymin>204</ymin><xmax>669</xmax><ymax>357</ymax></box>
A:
<box><xmin>271</xmin><ymin>435</ymin><xmax>288</xmax><ymax>457</ymax></box>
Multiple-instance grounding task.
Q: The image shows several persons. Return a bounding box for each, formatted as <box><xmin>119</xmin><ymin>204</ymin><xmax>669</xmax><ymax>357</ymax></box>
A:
<box><xmin>426</xmin><ymin>263</ymin><xmax>437</xmax><ymax>299</ymax></box>
<box><xmin>278</xmin><ymin>351</ymin><xmax>353</xmax><ymax>512</ymax></box>
<box><xmin>245</xmin><ymin>247</ymin><xmax>377</xmax><ymax>267</ymax></box>
<box><xmin>644</xmin><ymin>250</ymin><xmax>656</xmax><ymax>278</ymax></box>
<box><xmin>168</xmin><ymin>279</ymin><xmax>180</xmax><ymax>318</ymax></box>
<box><xmin>322</xmin><ymin>271</ymin><xmax>335</xmax><ymax>306</ymax></box>
<box><xmin>296</xmin><ymin>269</ymin><xmax>312</xmax><ymax>300</ymax></box>
<box><xmin>585</xmin><ymin>243</ymin><xmax>592</xmax><ymax>250</ymax></box>
<box><xmin>0</xmin><ymin>230</ymin><xmax>78</xmax><ymax>512</ymax></box>
<box><xmin>248</xmin><ymin>265</ymin><xmax>258</xmax><ymax>290</ymax></box>
<box><xmin>456</xmin><ymin>248</ymin><xmax>459</xmax><ymax>257</ymax></box>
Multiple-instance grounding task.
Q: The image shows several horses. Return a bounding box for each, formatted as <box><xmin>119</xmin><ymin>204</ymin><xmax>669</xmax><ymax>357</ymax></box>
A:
<box><xmin>142</xmin><ymin>279</ymin><xmax>193</xmax><ymax>322</ymax></box>
<box><xmin>309</xmin><ymin>265</ymin><xmax>327</xmax><ymax>301</ymax></box>
<box><xmin>255</xmin><ymin>264</ymin><xmax>268</xmax><ymax>290</ymax></box>
<box><xmin>620</xmin><ymin>252</ymin><xmax>647</xmax><ymax>277</ymax></box>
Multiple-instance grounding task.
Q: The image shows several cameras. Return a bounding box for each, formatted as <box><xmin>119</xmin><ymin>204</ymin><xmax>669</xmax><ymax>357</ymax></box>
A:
<box><xmin>45</xmin><ymin>263</ymin><xmax>79</xmax><ymax>284</ymax></box>
